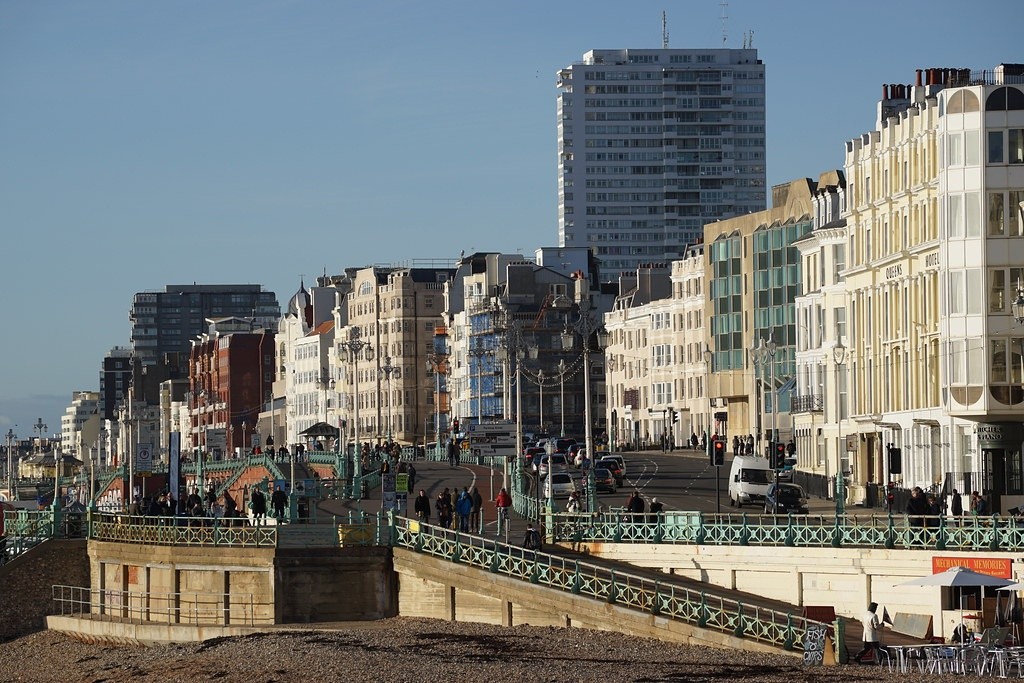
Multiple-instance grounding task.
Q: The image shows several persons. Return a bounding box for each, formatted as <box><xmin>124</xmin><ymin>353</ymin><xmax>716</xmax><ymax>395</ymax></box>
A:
<box><xmin>125</xmin><ymin>436</ymin><xmax>542</xmax><ymax>550</ymax></box>
<box><xmin>568</xmin><ymin>430</ymin><xmax>795</xmax><ymax>538</ymax></box>
<box><xmin>951</xmin><ymin>623</ymin><xmax>970</xmax><ymax>643</ymax></box>
<box><xmin>855</xmin><ymin>602</ymin><xmax>885</xmax><ymax>663</ymax></box>
<box><xmin>908</xmin><ymin>486</ymin><xmax>988</xmax><ymax>544</ymax></box>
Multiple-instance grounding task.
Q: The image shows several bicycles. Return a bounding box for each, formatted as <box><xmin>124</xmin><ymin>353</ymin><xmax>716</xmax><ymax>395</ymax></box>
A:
<box><xmin>500</xmin><ymin>509</ymin><xmax>509</xmax><ymax>524</ymax></box>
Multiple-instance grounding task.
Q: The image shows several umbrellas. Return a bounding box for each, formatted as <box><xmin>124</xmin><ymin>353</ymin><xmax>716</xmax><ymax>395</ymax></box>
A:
<box><xmin>894</xmin><ymin>567</ymin><xmax>1024</xmax><ymax>648</ymax></box>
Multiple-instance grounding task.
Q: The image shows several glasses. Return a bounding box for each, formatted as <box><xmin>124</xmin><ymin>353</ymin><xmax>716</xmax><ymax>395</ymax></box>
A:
<box><xmin>439</xmin><ymin>494</ymin><xmax>443</xmax><ymax>496</ymax></box>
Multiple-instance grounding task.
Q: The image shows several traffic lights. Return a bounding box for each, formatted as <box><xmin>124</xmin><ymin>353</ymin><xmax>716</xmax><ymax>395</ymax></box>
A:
<box><xmin>713</xmin><ymin>440</ymin><xmax>724</xmax><ymax>466</ymax></box>
<box><xmin>453</xmin><ymin>420</ymin><xmax>459</xmax><ymax>434</ymax></box>
<box><xmin>775</xmin><ymin>443</ymin><xmax>785</xmax><ymax>468</ymax></box>
<box><xmin>672</xmin><ymin>411</ymin><xmax>678</xmax><ymax>424</ymax></box>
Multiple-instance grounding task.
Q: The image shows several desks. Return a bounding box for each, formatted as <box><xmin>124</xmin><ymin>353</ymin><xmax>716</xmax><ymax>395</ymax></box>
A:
<box><xmin>886</xmin><ymin>644</ymin><xmax>953</xmax><ymax>674</ymax></box>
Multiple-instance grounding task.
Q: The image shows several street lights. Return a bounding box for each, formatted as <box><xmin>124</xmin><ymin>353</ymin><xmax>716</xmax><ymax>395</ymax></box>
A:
<box><xmin>560</xmin><ymin>293</ymin><xmax>612</xmax><ymax>484</ymax></box>
<box><xmin>557</xmin><ymin>359</ymin><xmax>566</xmax><ymax>437</ymax></box>
<box><xmin>377</xmin><ymin>358</ymin><xmax>401</xmax><ymax>445</ymax></box>
<box><xmin>425</xmin><ymin>354</ymin><xmax>451</xmax><ymax>461</ymax></box>
<box><xmin>315</xmin><ymin>368</ymin><xmax>336</xmax><ymax>423</ymax></box>
<box><xmin>465</xmin><ymin>348</ymin><xmax>494</xmax><ymax>425</ymax></box>
<box><xmin>338</xmin><ymin>326</ymin><xmax>374</xmax><ymax>498</ymax></box>
<box><xmin>538</xmin><ymin>369</ymin><xmax>545</xmax><ymax>427</ymax></box>
<box><xmin>33</xmin><ymin>417</ymin><xmax>48</xmax><ymax>452</ymax></box>
<box><xmin>496</xmin><ymin>318</ymin><xmax>538</xmax><ymax>493</ymax></box>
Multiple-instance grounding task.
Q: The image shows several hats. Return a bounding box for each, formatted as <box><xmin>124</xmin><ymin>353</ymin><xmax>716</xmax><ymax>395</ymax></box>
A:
<box><xmin>652</xmin><ymin>498</ymin><xmax>658</xmax><ymax>503</ymax></box>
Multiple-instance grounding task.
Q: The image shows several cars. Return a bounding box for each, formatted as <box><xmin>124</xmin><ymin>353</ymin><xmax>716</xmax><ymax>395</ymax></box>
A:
<box><xmin>522</xmin><ymin>433</ymin><xmax>611</xmax><ymax>482</ymax></box>
<box><xmin>596</xmin><ymin>460</ymin><xmax>623</xmax><ymax>487</ymax></box>
<box><xmin>774</xmin><ymin>458</ymin><xmax>798</xmax><ymax>484</ymax></box>
<box><xmin>764</xmin><ymin>484</ymin><xmax>811</xmax><ymax>514</ymax></box>
<box><xmin>542</xmin><ymin>473</ymin><xmax>575</xmax><ymax>499</ymax></box>
<box><xmin>582</xmin><ymin>469</ymin><xmax>617</xmax><ymax>494</ymax></box>
<box><xmin>601</xmin><ymin>455</ymin><xmax>627</xmax><ymax>479</ymax></box>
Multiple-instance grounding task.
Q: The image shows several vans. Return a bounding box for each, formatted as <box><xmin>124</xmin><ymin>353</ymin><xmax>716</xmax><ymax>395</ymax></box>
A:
<box><xmin>728</xmin><ymin>456</ymin><xmax>774</xmax><ymax>508</ymax></box>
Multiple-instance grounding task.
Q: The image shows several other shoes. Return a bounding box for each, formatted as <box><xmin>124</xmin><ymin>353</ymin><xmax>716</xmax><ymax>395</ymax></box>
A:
<box><xmin>854</xmin><ymin>654</ymin><xmax>861</xmax><ymax>665</ymax></box>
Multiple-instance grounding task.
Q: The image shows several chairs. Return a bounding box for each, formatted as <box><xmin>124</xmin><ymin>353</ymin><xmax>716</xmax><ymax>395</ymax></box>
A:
<box><xmin>878</xmin><ymin>644</ymin><xmax>1024</xmax><ymax>679</ymax></box>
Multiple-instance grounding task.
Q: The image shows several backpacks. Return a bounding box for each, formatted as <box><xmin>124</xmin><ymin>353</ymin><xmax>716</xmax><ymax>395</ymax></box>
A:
<box><xmin>529</xmin><ymin>530</ymin><xmax>541</xmax><ymax>549</ymax></box>
